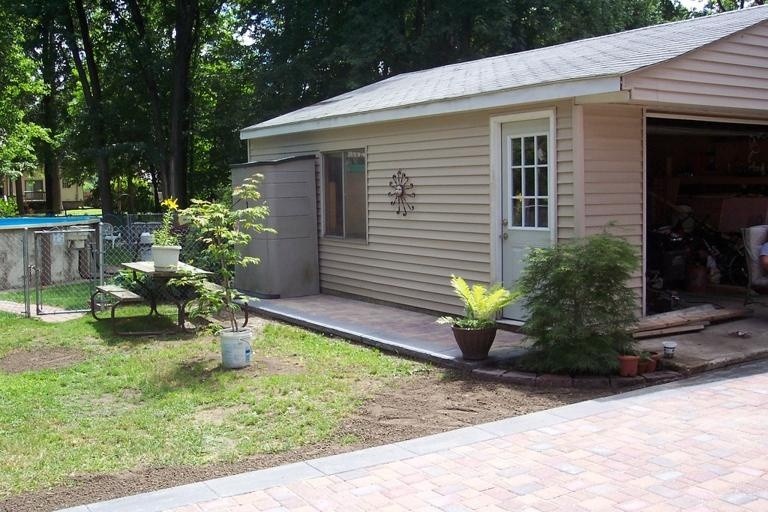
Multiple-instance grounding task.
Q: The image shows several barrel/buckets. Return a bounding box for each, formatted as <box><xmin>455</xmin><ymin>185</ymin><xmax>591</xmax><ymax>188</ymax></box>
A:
<box><xmin>220</xmin><ymin>327</ymin><xmax>254</xmax><ymax>369</ymax></box>
<box><xmin>662</xmin><ymin>341</ymin><xmax>678</xmax><ymax>358</ymax></box>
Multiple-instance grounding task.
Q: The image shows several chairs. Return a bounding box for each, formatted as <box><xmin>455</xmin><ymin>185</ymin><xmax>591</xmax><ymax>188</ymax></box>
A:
<box><xmin>102</xmin><ymin>222</ymin><xmax>122</xmax><ymax>251</ymax></box>
<box><xmin>741</xmin><ymin>223</ymin><xmax>767</xmax><ymax>308</ymax></box>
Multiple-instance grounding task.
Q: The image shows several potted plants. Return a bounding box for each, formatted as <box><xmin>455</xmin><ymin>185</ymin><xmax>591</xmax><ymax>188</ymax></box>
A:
<box><xmin>434</xmin><ymin>272</ymin><xmax>520</xmax><ymax>358</ymax></box>
<box><xmin>183</xmin><ymin>173</ymin><xmax>278</xmax><ymax>369</ymax></box>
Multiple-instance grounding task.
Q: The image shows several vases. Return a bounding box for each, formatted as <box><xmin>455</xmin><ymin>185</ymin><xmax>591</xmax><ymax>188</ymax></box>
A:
<box><xmin>619</xmin><ymin>355</ymin><xmax>640</xmax><ymax>377</ymax></box>
<box><xmin>639</xmin><ymin>361</ymin><xmax>648</xmax><ymax>373</ymax></box>
<box><xmin>640</xmin><ymin>350</ymin><xmax>659</xmax><ymax>372</ymax></box>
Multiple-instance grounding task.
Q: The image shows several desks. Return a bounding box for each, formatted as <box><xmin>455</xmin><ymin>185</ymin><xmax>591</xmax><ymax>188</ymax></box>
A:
<box><xmin>117</xmin><ymin>258</ymin><xmax>216</xmax><ymax>333</ymax></box>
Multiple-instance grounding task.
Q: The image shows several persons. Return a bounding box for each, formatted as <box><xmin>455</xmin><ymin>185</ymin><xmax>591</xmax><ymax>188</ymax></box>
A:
<box><xmin>758</xmin><ymin>232</ymin><xmax>768</xmax><ymax>281</ymax></box>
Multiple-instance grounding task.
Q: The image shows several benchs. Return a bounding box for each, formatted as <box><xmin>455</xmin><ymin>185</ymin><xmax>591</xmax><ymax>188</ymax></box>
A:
<box><xmin>90</xmin><ymin>284</ymin><xmax>155</xmax><ymax>335</ymax></box>
<box><xmin>196</xmin><ymin>282</ymin><xmax>248</xmax><ymax>329</ymax></box>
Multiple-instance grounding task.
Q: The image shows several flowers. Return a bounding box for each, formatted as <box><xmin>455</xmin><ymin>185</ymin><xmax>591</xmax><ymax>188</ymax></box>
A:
<box><xmin>150</xmin><ymin>197</ymin><xmax>179</xmax><ymax>245</ymax></box>
